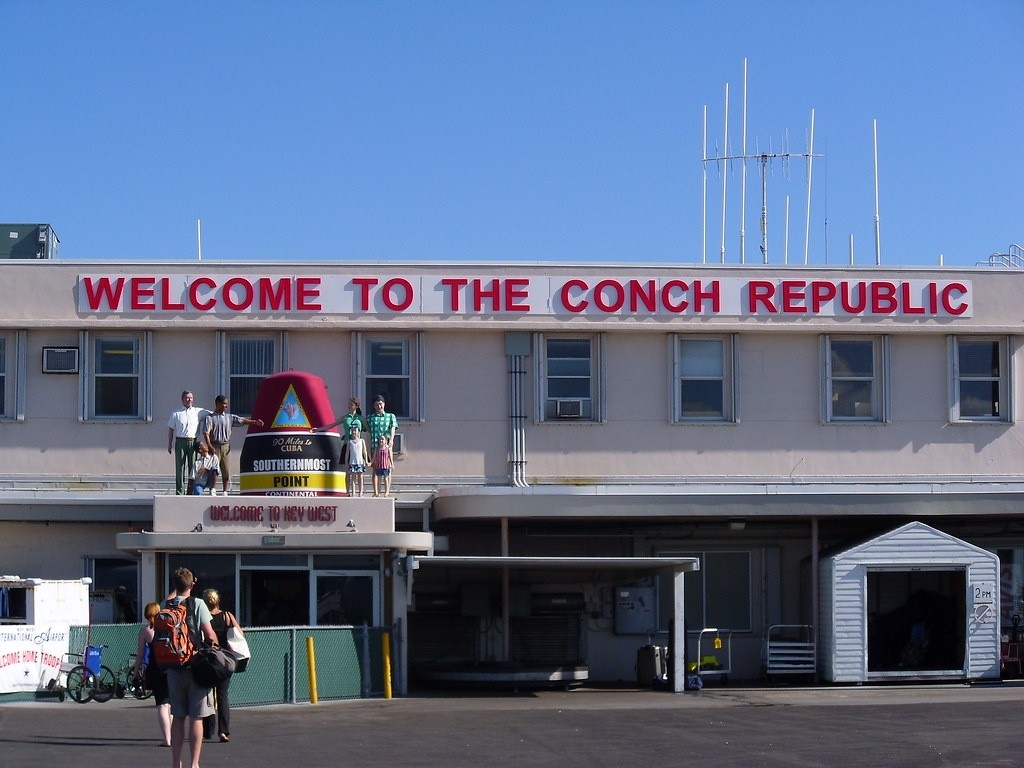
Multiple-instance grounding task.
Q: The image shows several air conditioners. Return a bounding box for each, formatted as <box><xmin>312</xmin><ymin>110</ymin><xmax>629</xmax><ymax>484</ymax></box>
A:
<box><xmin>39</xmin><ymin>346</ymin><xmax>80</xmax><ymax>376</ymax></box>
<box><xmin>389</xmin><ymin>431</ymin><xmax>403</xmax><ymax>454</ymax></box>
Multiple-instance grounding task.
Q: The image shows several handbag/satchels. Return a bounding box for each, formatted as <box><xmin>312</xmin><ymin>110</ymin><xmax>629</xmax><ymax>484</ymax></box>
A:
<box><xmin>226</xmin><ymin>626</ymin><xmax>251</xmax><ymax>675</ymax></box>
<box><xmin>191</xmin><ymin>645</ymin><xmax>237</xmax><ymax>688</ymax></box>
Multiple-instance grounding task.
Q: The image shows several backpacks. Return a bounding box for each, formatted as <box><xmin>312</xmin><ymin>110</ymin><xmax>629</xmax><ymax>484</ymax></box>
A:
<box><xmin>152</xmin><ymin>603</ymin><xmax>195</xmax><ymax>670</ymax></box>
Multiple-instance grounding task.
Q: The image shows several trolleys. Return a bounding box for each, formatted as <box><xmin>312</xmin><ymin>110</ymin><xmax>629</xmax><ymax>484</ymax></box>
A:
<box><xmin>758</xmin><ymin>620</ymin><xmax>818</xmax><ymax>686</ymax></box>
<box><xmin>686</xmin><ymin>628</ymin><xmax>733</xmax><ymax>683</ymax></box>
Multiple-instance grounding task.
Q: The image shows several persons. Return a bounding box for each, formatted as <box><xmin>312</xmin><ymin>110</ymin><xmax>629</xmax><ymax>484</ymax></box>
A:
<box><xmin>309</xmin><ymin>397</ymin><xmax>367</xmax><ymax>497</ymax></box>
<box><xmin>132</xmin><ymin>601</ymin><xmax>174</xmax><ymax>748</ymax></box>
<box><xmin>368</xmin><ymin>435</ymin><xmax>395</xmax><ymax>497</ymax></box>
<box><xmin>167</xmin><ymin>390</ymin><xmax>251</xmax><ymax>495</ymax></box>
<box><xmin>365</xmin><ymin>395</ymin><xmax>399</xmax><ymax>497</ymax></box>
<box><xmin>189</xmin><ymin>441</ymin><xmax>219</xmax><ymax>496</ymax></box>
<box><xmin>203</xmin><ymin>394</ymin><xmax>264</xmax><ymax>497</ymax></box>
<box><xmin>201</xmin><ymin>587</ymin><xmax>244</xmax><ymax>743</ymax></box>
<box><xmin>345</xmin><ymin>425</ymin><xmax>367</xmax><ymax>496</ymax></box>
<box><xmin>152</xmin><ymin>567</ymin><xmax>219</xmax><ymax>768</ymax></box>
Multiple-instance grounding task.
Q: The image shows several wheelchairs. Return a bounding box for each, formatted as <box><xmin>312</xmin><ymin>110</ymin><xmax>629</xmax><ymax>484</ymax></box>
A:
<box><xmin>125</xmin><ymin>643</ymin><xmax>157</xmax><ymax>699</ymax></box>
<box><xmin>46</xmin><ymin>644</ymin><xmax>117</xmax><ymax>704</ymax></box>
